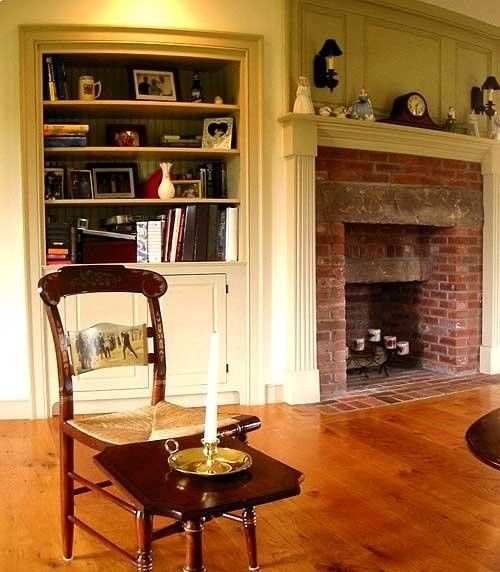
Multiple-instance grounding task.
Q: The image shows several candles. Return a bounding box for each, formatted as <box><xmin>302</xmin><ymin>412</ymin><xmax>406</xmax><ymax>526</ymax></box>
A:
<box><xmin>203</xmin><ymin>331</ymin><xmax>219</xmax><ymax>442</ymax></box>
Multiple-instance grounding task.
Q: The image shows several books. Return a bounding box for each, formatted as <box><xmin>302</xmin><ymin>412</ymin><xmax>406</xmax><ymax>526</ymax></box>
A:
<box><xmin>45</xmin><ymin>56</ymin><xmax>72</xmax><ymax>102</ymax></box>
<box><xmin>159</xmin><ymin>133</ymin><xmax>202</xmax><ymax>148</ymax></box>
<box><xmin>156</xmin><ymin>202</ymin><xmax>239</xmax><ymax>264</ymax></box>
<box><xmin>196</xmin><ymin>161</ymin><xmax>227</xmax><ymax>198</ymax></box>
<box><xmin>82</xmin><ymin>214</ymin><xmax>150</xmax><ymax>240</ymax></box>
<box><xmin>44</xmin><ymin>122</ymin><xmax>90</xmax><ymax>147</ymax></box>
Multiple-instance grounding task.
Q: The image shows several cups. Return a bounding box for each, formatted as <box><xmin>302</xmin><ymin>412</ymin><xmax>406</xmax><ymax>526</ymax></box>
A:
<box><xmin>78</xmin><ymin>75</ymin><xmax>101</xmax><ymax>101</ymax></box>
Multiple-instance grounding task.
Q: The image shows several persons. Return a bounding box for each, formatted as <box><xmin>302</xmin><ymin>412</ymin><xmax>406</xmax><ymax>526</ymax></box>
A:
<box><xmin>80</xmin><ymin>176</ymin><xmax>90</xmax><ymax>197</ymax></box>
<box><xmin>157</xmin><ymin>75</ymin><xmax>172</xmax><ymax>96</ymax></box>
<box><xmin>444</xmin><ymin>105</ymin><xmax>457</xmax><ymax>134</ymax></box>
<box><xmin>213</xmin><ymin>128</ymin><xmax>225</xmax><ymax>139</ymax></box>
<box><xmin>149</xmin><ymin>78</ymin><xmax>161</xmax><ymax>96</ymax></box>
<box><xmin>107</xmin><ymin>174</ymin><xmax>119</xmax><ymax>193</ymax></box>
<box><xmin>74</xmin><ymin>330</ymin><xmax>121</xmax><ymax>369</ymax></box>
<box><xmin>467</xmin><ymin>108</ymin><xmax>481</xmax><ymax>138</ymax></box>
<box><xmin>293</xmin><ymin>74</ymin><xmax>376</xmax><ymax>122</ymax></box>
<box><xmin>119</xmin><ymin>331</ymin><xmax>139</xmax><ymax>360</ymax></box>
<box><xmin>139</xmin><ymin>75</ymin><xmax>151</xmax><ymax>94</ymax></box>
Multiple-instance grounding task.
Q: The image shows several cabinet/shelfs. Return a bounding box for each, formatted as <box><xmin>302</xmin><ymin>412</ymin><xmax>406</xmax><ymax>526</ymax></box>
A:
<box><xmin>63</xmin><ymin>274</ymin><xmax>228</xmax><ymax>392</ymax></box>
<box><xmin>34</xmin><ymin>40</ymin><xmax>251</xmax><ymax>265</ymax></box>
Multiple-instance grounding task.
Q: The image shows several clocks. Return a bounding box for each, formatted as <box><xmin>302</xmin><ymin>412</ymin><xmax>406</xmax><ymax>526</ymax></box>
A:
<box><xmin>375</xmin><ymin>91</ymin><xmax>450</xmax><ymax>131</ymax></box>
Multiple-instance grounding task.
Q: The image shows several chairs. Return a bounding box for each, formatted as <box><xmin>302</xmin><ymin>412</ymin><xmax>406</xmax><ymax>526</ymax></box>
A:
<box><xmin>36</xmin><ymin>263</ymin><xmax>261</xmax><ymax>572</ymax></box>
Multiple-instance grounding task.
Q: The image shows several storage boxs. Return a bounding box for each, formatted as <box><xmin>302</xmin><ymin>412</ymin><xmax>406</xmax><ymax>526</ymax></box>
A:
<box><xmin>78</xmin><ymin>232</ymin><xmax>137</xmax><ymax>264</ymax></box>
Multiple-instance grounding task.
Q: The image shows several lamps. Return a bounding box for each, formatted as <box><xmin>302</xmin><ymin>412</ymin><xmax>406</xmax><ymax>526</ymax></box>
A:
<box><xmin>471</xmin><ymin>76</ymin><xmax>500</xmax><ymax>116</ymax></box>
<box><xmin>314</xmin><ymin>39</ymin><xmax>343</xmax><ymax>92</ymax></box>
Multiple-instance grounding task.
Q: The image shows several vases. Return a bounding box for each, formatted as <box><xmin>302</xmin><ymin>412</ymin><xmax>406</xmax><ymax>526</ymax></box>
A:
<box><xmin>157</xmin><ymin>161</ymin><xmax>176</xmax><ymax>198</ymax></box>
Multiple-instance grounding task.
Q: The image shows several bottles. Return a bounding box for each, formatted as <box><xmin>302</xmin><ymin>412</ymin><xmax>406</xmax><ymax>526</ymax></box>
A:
<box><xmin>191</xmin><ymin>71</ymin><xmax>203</xmax><ymax>103</ymax></box>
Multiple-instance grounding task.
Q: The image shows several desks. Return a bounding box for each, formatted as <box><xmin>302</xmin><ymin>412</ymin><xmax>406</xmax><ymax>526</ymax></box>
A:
<box><xmin>465</xmin><ymin>408</ymin><xmax>499</xmax><ymax>471</ymax></box>
<box><xmin>93</xmin><ymin>430</ymin><xmax>304</xmax><ymax>571</ymax></box>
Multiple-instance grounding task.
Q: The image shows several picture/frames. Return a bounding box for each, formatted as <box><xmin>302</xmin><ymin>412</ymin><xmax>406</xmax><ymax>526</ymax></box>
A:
<box><xmin>202</xmin><ymin>116</ymin><xmax>233</xmax><ymax>149</ymax></box>
<box><xmin>44</xmin><ymin>168</ymin><xmax>65</xmax><ymax>200</ymax></box>
<box><xmin>133</xmin><ymin>69</ymin><xmax>176</xmax><ymax>101</ymax></box>
<box><xmin>67</xmin><ymin>168</ymin><xmax>93</xmax><ymax>199</ymax></box>
<box><xmin>92</xmin><ymin>168</ymin><xmax>135</xmax><ymax>199</ymax></box>
